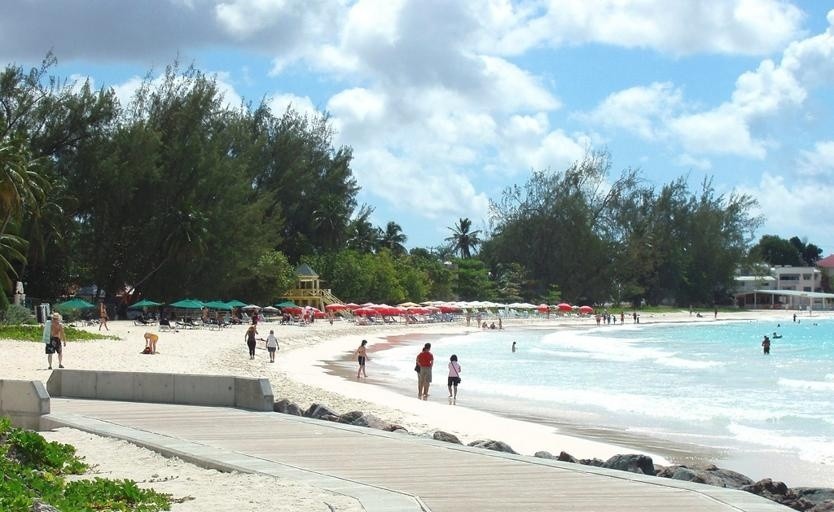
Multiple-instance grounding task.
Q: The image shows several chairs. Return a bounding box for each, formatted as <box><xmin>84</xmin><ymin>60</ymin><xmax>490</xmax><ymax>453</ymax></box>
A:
<box><xmin>123</xmin><ymin>294</ymin><xmax>594</xmax><ymax>333</ymax></box>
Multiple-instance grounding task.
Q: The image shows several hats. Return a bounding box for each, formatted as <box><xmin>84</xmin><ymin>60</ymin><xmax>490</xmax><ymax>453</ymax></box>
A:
<box><xmin>50</xmin><ymin>313</ymin><xmax>61</xmax><ymax>318</ymax></box>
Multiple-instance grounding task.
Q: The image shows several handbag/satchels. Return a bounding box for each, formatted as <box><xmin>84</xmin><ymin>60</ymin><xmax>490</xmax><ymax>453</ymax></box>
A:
<box><xmin>458</xmin><ymin>378</ymin><xmax>461</xmax><ymax>382</ymax></box>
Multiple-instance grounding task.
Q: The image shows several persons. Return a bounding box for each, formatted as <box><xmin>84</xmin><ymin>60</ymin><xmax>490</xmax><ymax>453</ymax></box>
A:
<box><xmin>595</xmin><ymin>311</ymin><xmax>640</xmax><ymax>324</ymax></box>
<box><xmin>266</xmin><ymin>330</ymin><xmax>279</xmax><ymax>363</ymax></box>
<box><xmin>773</xmin><ymin>332</ymin><xmax>781</xmax><ymax>338</ymax></box>
<box><xmin>714</xmin><ymin>306</ymin><xmax>718</xmax><ymax>317</ymax></box>
<box><xmin>690</xmin><ymin>305</ymin><xmax>703</xmax><ymax>317</ymax></box>
<box><xmin>794</xmin><ymin>314</ymin><xmax>797</xmax><ymax>321</ymax></box>
<box><xmin>353</xmin><ymin>340</ymin><xmax>369</xmax><ymax>378</ymax></box>
<box><xmin>762</xmin><ymin>336</ymin><xmax>770</xmax><ymax>354</ymax></box>
<box><xmin>512</xmin><ymin>342</ymin><xmax>516</xmax><ymax>352</ymax></box>
<box><xmin>447</xmin><ymin>355</ymin><xmax>461</xmax><ymax>398</ymax></box>
<box><xmin>245</xmin><ymin>324</ymin><xmax>258</xmax><ymax>360</ymax></box>
<box><xmin>43</xmin><ymin>312</ymin><xmax>67</xmax><ymax>369</ymax></box>
<box><xmin>143</xmin><ymin>331</ymin><xmax>158</xmax><ymax>356</ymax></box>
<box><xmin>416</xmin><ymin>343</ymin><xmax>434</xmax><ymax>396</ymax></box>
